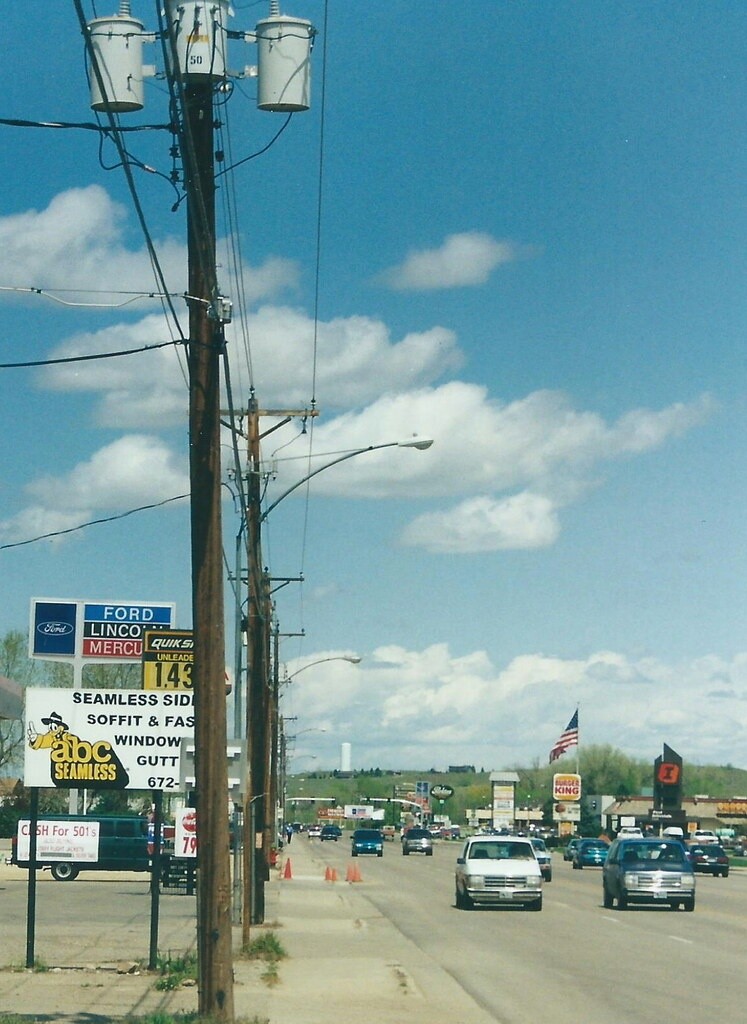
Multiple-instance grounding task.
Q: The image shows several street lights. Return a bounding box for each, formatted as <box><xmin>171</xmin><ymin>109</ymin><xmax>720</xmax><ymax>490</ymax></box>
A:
<box><xmin>282</xmin><ymin>857</ymin><xmax>293</xmax><ymax>880</ymax></box>
<box><xmin>265</xmin><ymin>655</ymin><xmax>362</xmax><ymax>853</ymax></box>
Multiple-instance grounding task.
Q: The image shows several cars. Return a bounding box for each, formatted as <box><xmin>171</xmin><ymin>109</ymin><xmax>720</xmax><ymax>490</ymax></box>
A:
<box><xmin>350</xmin><ymin>828</ymin><xmax>385</xmax><ymax>857</ymax></box>
<box><xmin>400</xmin><ymin>828</ymin><xmax>435</xmax><ymax>856</ymax></box>
<box><xmin>689</xmin><ymin>844</ymin><xmax>730</xmax><ymax>878</ymax></box>
<box><xmin>617</xmin><ymin>827</ymin><xmax>644</xmax><ymax>841</ymax></box>
<box><xmin>563</xmin><ymin>836</ymin><xmax>611</xmax><ymax>869</ymax></box>
<box><xmin>690</xmin><ymin>829</ymin><xmax>720</xmax><ymax>845</ymax></box>
<box><xmin>292</xmin><ymin>821</ymin><xmax>343</xmax><ymax>842</ymax></box>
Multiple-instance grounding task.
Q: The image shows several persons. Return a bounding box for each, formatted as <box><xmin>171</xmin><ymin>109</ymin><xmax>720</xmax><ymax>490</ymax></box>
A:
<box><xmin>287</xmin><ymin>823</ymin><xmax>293</xmax><ymax>844</ymax></box>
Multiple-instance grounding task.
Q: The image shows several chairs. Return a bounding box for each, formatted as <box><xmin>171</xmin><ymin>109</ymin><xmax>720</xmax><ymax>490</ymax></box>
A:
<box><xmin>624</xmin><ymin>851</ymin><xmax>639</xmax><ymax>860</ymax></box>
<box><xmin>509</xmin><ymin>844</ymin><xmax>529</xmax><ymax>857</ymax></box>
<box><xmin>658</xmin><ymin>849</ymin><xmax>682</xmax><ymax>863</ymax></box>
<box><xmin>474</xmin><ymin>849</ymin><xmax>489</xmax><ymax>859</ymax></box>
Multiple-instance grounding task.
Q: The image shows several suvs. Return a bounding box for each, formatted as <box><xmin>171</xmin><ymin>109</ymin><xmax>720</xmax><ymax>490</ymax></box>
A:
<box><xmin>602</xmin><ymin>836</ymin><xmax>697</xmax><ymax>912</ymax></box>
<box><xmin>456</xmin><ymin>834</ymin><xmax>546</xmax><ymax>911</ymax></box>
<box><xmin>526</xmin><ymin>837</ymin><xmax>554</xmax><ymax>882</ymax></box>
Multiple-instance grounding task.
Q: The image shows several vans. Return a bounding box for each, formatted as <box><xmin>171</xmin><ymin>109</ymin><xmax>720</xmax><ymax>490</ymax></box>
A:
<box><xmin>10</xmin><ymin>813</ymin><xmax>174</xmax><ymax>882</ymax></box>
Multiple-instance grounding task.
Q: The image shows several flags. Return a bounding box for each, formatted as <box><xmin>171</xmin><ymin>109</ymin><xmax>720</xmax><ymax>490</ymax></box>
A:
<box><xmin>549</xmin><ymin>708</ymin><xmax>578</xmax><ymax>764</ymax></box>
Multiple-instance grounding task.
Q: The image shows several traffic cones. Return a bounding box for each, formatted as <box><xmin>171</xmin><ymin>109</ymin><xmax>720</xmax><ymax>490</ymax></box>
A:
<box><xmin>244</xmin><ymin>437</ymin><xmax>437</xmax><ymax>925</ymax></box>
<box><xmin>324</xmin><ymin>866</ymin><xmax>332</xmax><ymax>880</ymax></box>
<box><xmin>330</xmin><ymin>867</ymin><xmax>338</xmax><ymax>881</ymax></box>
<box><xmin>345</xmin><ymin>865</ymin><xmax>355</xmax><ymax>881</ymax></box>
<box><xmin>352</xmin><ymin>863</ymin><xmax>361</xmax><ymax>882</ymax></box>
